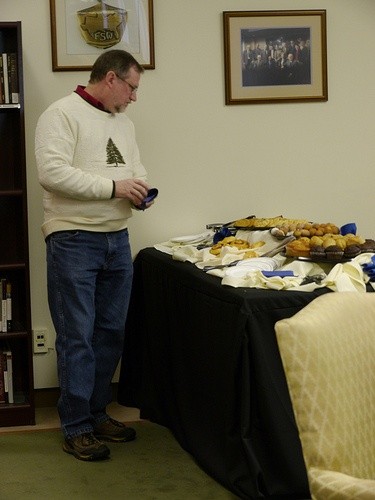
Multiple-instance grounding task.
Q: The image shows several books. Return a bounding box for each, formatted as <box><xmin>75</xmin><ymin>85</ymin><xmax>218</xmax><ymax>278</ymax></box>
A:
<box><xmin>0</xmin><ymin>53</ymin><xmax>19</xmax><ymax>105</ymax></box>
<box><xmin>0</xmin><ymin>278</ymin><xmax>14</xmax><ymax>404</ymax></box>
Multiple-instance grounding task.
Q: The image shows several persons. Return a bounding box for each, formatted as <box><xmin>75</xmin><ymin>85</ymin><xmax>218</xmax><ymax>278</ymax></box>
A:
<box><xmin>35</xmin><ymin>49</ymin><xmax>155</xmax><ymax>461</ymax></box>
<box><xmin>244</xmin><ymin>37</ymin><xmax>310</xmax><ymax>79</ymax></box>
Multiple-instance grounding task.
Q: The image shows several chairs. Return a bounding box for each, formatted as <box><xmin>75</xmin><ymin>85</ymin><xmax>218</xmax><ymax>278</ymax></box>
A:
<box><xmin>275</xmin><ymin>292</ymin><xmax>375</xmax><ymax>500</ymax></box>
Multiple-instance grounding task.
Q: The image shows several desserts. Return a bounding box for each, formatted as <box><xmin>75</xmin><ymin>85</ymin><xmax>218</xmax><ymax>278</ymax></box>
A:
<box><xmin>270</xmin><ymin>222</ymin><xmax>375</xmax><ymax>260</ymax></box>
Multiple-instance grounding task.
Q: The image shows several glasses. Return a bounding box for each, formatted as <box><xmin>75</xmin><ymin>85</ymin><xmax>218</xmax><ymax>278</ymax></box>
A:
<box><xmin>116</xmin><ymin>74</ymin><xmax>137</xmax><ymax>94</ymax></box>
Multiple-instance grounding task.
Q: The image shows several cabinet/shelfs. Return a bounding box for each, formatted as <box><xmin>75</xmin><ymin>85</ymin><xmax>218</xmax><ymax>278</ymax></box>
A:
<box><xmin>0</xmin><ymin>21</ymin><xmax>36</xmax><ymax>427</ymax></box>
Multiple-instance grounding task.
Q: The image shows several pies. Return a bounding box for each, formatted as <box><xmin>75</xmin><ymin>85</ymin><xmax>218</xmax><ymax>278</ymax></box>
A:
<box><xmin>209</xmin><ymin>217</ymin><xmax>308</xmax><ymax>255</ymax></box>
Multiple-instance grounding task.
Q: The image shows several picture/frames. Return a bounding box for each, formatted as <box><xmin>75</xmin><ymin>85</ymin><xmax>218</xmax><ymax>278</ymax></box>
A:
<box><xmin>49</xmin><ymin>0</ymin><xmax>155</xmax><ymax>72</ymax></box>
<box><xmin>223</xmin><ymin>10</ymin><xmax>328</xmax><ymax>105</ymax></box>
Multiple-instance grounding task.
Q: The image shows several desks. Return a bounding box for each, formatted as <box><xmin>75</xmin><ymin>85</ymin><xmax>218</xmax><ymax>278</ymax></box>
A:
<box><xmin>118</xmin><ymin>241</ymin><xmax>334</xmax><ymax>500</ymax></box>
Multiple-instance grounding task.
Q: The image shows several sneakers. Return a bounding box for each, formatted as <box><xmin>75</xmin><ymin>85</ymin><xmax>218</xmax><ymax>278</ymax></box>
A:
<box><xmin>94</xmin><ymin>415</ymin><xmax>136</xmax><ymax>442</ymax></box>
<box><xmin>63</xmin><ymin>435</ymin><xmax>111</xmax><ymax>461</ymax></box>
